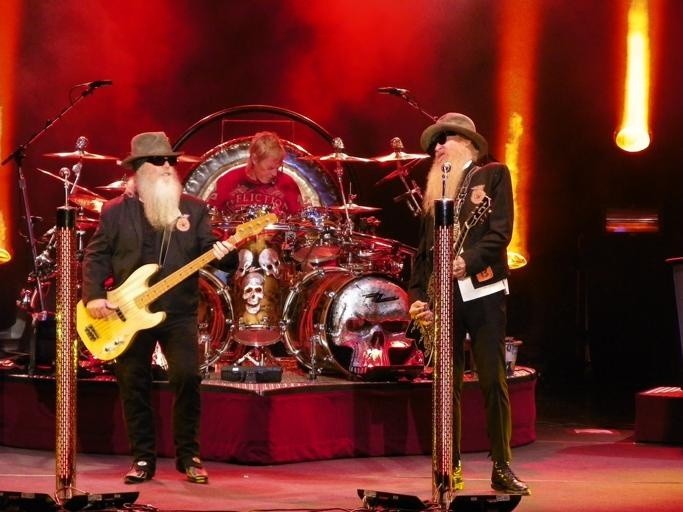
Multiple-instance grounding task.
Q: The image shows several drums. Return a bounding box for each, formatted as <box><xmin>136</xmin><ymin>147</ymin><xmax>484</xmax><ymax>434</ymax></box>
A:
<box><xmin>281</xmin><ymin>267</ymin><xmax>411</xmax><ymax>380</ymax></box>
<box><xmin>225</xmin><ymin>267</ymin><xmax>287</xmax><ymax>348</ymax></box>
<box><xmin>291</xmin><ymin>243</ymin><xmax>342</xmax><ymax>271</ymax></box>
<box><xmin>151</xmin><ymin>270</ymin><xmax>237</xmax><ymax>370</ymax></box>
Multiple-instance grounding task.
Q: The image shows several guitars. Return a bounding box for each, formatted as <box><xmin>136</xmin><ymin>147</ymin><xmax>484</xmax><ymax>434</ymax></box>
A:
<box><xmin>76</xmin><ymin>213</ymin><xmax>278</xmax><ymax>361</ymax></box>
<box><xmin>413</xmin><ymin>196</ymin><xmax>494</xmax><ymax>375</ymax></box>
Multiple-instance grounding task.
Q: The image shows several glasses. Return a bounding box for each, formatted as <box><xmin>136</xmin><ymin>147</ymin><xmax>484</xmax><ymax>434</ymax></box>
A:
<box><xmin>143</xmin><ymin>156</ymin><xmax>176</xmax><ymax>167</ymax></box>
<box><xmin>425</xmin><ymin>130</ymin><xmax>457</xmax><ymax>153</ymax></box>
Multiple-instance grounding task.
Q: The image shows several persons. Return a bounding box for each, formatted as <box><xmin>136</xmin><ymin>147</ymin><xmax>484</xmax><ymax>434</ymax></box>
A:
<box><xmin>409</xmin><ymin>113</ymin><xmax>531</xmax><ymax>496</ymax></box>
<box><xmin>83</xmin><ymin>131</ymin><xmax>239</xmax><ymax>483</ymax></box>
<box><xmin>204</xmin><ymin>132</ymin><xmax>304</xmax><ymax>358</ymax></box>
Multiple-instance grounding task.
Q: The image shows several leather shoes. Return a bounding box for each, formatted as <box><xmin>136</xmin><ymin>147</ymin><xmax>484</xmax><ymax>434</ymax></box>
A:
<box><xmin>174</xmin><ymin>457</ymin><xmax>208</xmax><ymax>483</ymax></box>
<box><xmin>123</xmin><ymin>461</ymin><xmax>154</xmax><ymax>483</ymax></box>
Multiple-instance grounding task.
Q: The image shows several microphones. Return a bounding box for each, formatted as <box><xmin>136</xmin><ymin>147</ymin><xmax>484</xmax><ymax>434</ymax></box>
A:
<box><xmin>85</xmin><ymin>78</ymin><xmax>112</xmax><ymax>86</ymax></box>
<box><xmin>441</xmin><ymin>162</ymin><xmax>455</xmax><ymax>178</ymax></box>
<box><xmin>377</xmin><ymin>87</ymin><xmax>407</xmax><ymax>95</ymax></box>
<box><xmin>54</xmin><ymin>164</ymin><xmax>74</xmax><ymax>183</ymax></box>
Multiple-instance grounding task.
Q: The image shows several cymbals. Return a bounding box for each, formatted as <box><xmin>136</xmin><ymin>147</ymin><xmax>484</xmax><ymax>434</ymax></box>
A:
<box><xmin>327</xmin><ymin>195</ymin><xmax>383</xmax><ymax>214</ymax></box>
<box><xmin>264</xmin><ymin>222</ymin><xmax>322</xmax><ymax>231</ymax></box>
<box><xmin>298</xmin><ymin>137</ymin><xmax>372</xmax><ymax>167</ymax></box>
<box><xmin>373</xmin><ymin>136</ymin><xmax>431</xmax><ymax>163</ymax></box>
<box><xmin>95</xmin><ymin>180</ymin><xmax>128</xmax><ymax>192</ymax></box>
<box><xmin>73</xmin><ymin>214</ymin><xmax>100</xmax><ymax>230</ymax></box>
<box><xmin>68</xmin><ymin>194</ymin><xmax>107</xmax><ymax>214</ymax></box>
<box><xmin>44</xmin><ymin>135</ymin><xmax>119</xmax><ymax>162</ymax></box>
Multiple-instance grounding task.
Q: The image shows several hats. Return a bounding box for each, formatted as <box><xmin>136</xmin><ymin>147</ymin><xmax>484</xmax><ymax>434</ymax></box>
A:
<box><xmin>120</xmin><ymin>132</ymin><xmax>184</xmax><ymax>169</ymax></box>
<box><xmin>419</xmin><ymin>112</ymin><xmax>488</xmax><ymax>159</ymax></box>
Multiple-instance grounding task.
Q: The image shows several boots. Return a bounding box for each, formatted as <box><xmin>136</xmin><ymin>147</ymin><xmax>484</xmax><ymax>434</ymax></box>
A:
<box><xmin>449</xmin><ymin>460</ymin><xmax>463</xmax><ymax>491</ymax></box>
<box><xmin>489</xmin><ymin>458</ymin><xmax>531</xmax><ymax>496</ymax></box>
